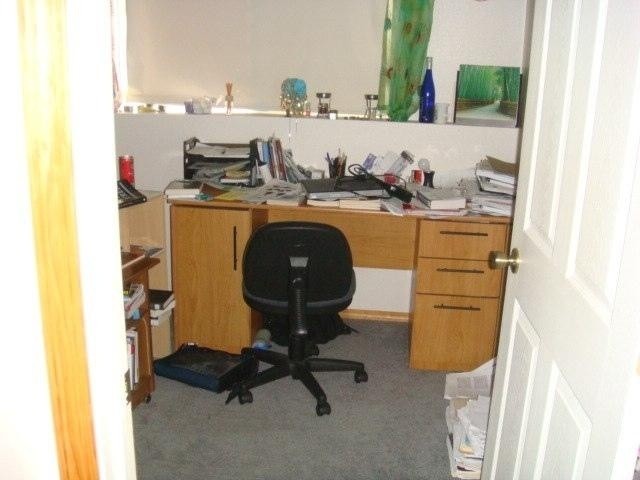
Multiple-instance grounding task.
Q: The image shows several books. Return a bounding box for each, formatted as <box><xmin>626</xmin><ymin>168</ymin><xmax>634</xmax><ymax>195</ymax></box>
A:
<box><xmin>124</xmin><ymin>283</ymin><xmax>175</xmax><ymax>395</ymax></box>
<box><xmin>443</xmin><ymin>358</ymin><xmax>498</xmax><ymax>478</ymax></box>
<box><xmin>163</xmin><ymin>135</ymin><xmax>517</xmax><ymax>216</ymax></box>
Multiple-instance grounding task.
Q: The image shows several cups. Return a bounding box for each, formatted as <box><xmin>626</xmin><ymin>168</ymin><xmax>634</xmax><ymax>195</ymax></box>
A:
<box><xmin>435</xmin><ymin>103</ymin><xmax>451</xmax><ymax>124</ymax></box>
<box><xmin>119</xmin><ymin>155</ymin><xmax>135</xmax><ymax>189</ymax></box>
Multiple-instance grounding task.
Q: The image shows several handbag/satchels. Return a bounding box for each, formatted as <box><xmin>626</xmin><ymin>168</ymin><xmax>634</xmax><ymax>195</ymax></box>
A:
<box><xmin>152</xmin><ymin>343</ymin><xmax>259</xmax><ymax>394</ymax></box>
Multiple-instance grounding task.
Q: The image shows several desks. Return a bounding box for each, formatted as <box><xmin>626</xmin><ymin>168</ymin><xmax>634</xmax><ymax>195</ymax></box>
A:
<box><xmin>166</xmin><ymin>184</ymin><xmax>515</xmax><ymax>378</ymax></box>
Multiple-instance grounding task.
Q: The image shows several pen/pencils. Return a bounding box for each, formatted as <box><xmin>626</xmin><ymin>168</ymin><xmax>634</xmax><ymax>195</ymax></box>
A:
<box><xmin>325</xmin><ymin>147</ymin><xmax>348</xmax><ymax>180</ymax></box>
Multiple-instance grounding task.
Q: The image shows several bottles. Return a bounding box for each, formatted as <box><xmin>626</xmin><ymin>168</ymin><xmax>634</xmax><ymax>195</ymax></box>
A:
<box><xmin>422</xmin><ymin>171</ymin><xmax>435</xmax><ymax>189</ymax></box>
<box><xmin>418</xmin><ymin>57</ymin><xmax>435</xmax><ymax>121</ymax></box>
<box><xmin>366</xmin><ymin>95</ymin><xmax>378</xmax><ymax>120</ymax></box>
<box><xmin>253</xmin><ymin>329</ymin><xmax>270</xmax><ymax>350</ymax></box>
<box><xmin>317</xmin><ymin>93</ymin><xmax>331</xmax><ymax>117</ymax></box>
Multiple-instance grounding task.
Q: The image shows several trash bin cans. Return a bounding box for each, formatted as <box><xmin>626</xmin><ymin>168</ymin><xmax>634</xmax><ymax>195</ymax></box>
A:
<box><xmin>150</xmin><ymin>289</ymin><xmax>175</xmax><ymax>360</ymax></box>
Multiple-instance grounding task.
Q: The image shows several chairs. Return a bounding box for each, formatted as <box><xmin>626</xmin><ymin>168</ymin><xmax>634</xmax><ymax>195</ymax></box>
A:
<box><xmin>236</xmin><ymin>222</ymin><xmax>369</xmax><ymax>416</ymax></box>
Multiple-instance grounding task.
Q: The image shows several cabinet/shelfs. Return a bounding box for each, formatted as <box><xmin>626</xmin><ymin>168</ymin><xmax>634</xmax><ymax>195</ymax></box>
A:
<box><xmin>118</xmin><ymin>184</ymin><xmax>172</xmax><ymax>407</ymax></box>
